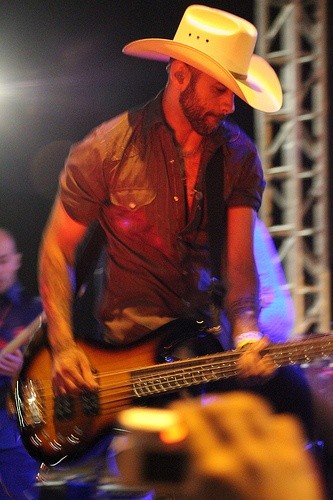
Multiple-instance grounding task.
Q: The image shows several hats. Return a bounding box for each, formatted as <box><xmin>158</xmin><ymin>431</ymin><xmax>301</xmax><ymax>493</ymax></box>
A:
<box><xmin>121</xmin><ymin>5</ymin><xmax>283</xmax><ymax>113</ymax></box>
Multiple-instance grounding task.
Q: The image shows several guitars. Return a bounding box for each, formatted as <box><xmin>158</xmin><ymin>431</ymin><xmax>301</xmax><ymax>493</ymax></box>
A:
<box><xmin>6</xmin><ymin>320</ymin><xmax>333</xmax><ymax>470</ymax></box>
<box><xmin>0</xmin><ymin>308</ymin><xmax>48</xmax><ymax>362</ymax></box>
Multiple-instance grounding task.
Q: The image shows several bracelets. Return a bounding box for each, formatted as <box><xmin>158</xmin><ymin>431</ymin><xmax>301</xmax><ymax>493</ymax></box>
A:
<box><xmin>226</xmin><ymin>296</ymin><xmax>257</xmax><ymax>315</ymax></box>
<box><xmin>233</xmin><ymin>332</ymin><xmax>269</xmax><ymax>351</ymax></box>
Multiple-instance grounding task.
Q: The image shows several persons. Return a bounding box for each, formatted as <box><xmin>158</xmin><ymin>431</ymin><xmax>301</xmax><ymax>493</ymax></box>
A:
<box><xmin>181</xmin><ymin>392</ymin><xmax>329</xmax><ymax>500</ymax></box>
<box><xmin>213</xmin><ymin>209</ymin><xmax>293</xmax><ymax>355</ymax></box>
<box><xmin>40</xmin><ymin>1</ymin><xmax>283</xmax><ymax>500</ymax></box>
<box><xmin>0</xmin><ymin>228</ymin><xmax>46</xmax><ymax>500</ymax></box>
